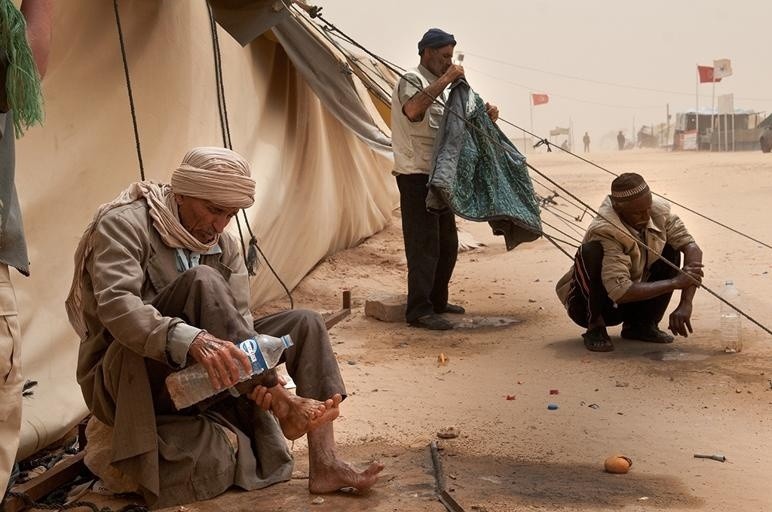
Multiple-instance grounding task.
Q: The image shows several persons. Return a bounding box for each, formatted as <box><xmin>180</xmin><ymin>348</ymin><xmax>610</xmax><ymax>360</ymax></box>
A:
<box><xmin>64</xmin><ymin>146</ymin><xmax>385</xmax><ymax>494</ymax></box>
<box><xmin>387</xmin><ymin>27</ymin><xmax>500</xmax><ymax>331</ymax></box>
<box><xmin>561</xmin><ymin>139</ymin><xmax>568</xmax><ymax>150</ymax></box>
<box><xmin>617</xmin><ymin>131</ymin><xmax>625</xmax><ymax>151</ymax></box>
<box><xmin>555</xmin><ymin>172</ymin><xmax>703</xmax><ymax>353</ymax></box>
<box><xmin>582</xmin><ymin>131</ymin><xmax>590</xmax><ymax>153</ymax></box>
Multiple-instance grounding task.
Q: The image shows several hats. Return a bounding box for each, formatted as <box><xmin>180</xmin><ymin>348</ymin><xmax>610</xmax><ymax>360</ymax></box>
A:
<box><xmin>171</xmin><ymin>145</ymin><xmax>257</xmax><ymax>210</ymax></box>
<box><xmin>611</xmin><ymin>171</ymin><xmax>650</xmax><ymax>202</ymax></box>
<box><xmin>417</xmin><ymin>27</ymin><xmax>456</xmax><ymax>55</ymax></box>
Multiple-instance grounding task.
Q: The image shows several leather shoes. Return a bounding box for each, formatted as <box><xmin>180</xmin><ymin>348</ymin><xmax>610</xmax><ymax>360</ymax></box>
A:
<box><xmin>432</xmin><ymin>302</ymin><xmax>465</xmax><ymax>314</ymax></box>
<box><xmin>411</xmin><ymin>313</ymin><xmax>456</xmax><ymax>331</ymax></box>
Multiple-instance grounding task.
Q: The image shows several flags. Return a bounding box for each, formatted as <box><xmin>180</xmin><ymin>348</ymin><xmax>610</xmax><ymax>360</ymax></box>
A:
<box><xmin>697</xmin><ymin>66</ymin><xmax>721</xmax><ymax>83</ymax></box>
<box><xmin>532</xmin><ymin>94</ymin><xmax>549</xmax><ymax>106</ymax></box>
<box><xmin>713</xmin><ymin>57</ymin><xmax>732</xmax><ymax>79</ymax></box>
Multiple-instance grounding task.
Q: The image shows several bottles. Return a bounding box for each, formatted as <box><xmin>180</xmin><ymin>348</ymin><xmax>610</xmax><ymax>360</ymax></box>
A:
<box><xmin>164</xmin><ymin>332</ymin><xmax>294</xmax><ymax>408</ymax></box>
<box><xmin>719</xmin><ymin>281</ymin><xmax>741</xmax><ymax>353</ymax></box>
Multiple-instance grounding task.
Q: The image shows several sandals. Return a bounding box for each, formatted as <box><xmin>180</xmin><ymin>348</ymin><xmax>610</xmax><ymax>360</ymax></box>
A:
<box><xmin>622</xmin><ymin>326</ymin><xmax>675</xmax><ymax>344</ymax></box>
<box><xmin>583</xmin><ymin>330</ymin><xmax>615</xmax><ymax>353</ymax></box>
